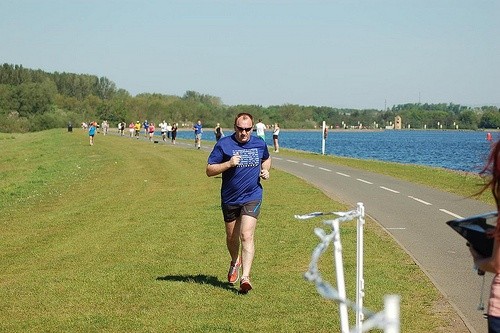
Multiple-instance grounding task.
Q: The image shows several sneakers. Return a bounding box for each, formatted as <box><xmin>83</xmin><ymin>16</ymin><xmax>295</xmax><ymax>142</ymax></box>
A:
<box><xmin>228</xmin><ymin>256</ymin><xmax>253</xmax><ymax>289</ymax></box>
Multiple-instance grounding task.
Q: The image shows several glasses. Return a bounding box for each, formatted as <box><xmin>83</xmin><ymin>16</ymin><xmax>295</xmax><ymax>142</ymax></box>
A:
<box><xmin>235</xmin><ymin>125</ymin><xmax>254</xmax><ymax>133</ymax></box>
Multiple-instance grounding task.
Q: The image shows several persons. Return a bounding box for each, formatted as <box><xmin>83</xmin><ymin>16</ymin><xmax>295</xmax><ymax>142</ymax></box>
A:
<box><xmin>81</xmin><ymin>119</ymin><xmax>280</xmax><ymax>153</ymax></box>
<box><xmin>206</xmin><ymin>113</ymin><xmax>272</xmax><ymax>291</ymax></box>
<box><xmin>466</xmin><ymin>141</ymin><xmax>500</xmax><ymax>333</ymax></box>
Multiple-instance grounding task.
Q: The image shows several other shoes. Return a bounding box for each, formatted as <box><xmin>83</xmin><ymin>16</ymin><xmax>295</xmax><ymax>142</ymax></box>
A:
<box><xmin>273</xmin><ymin>150</ymin><xmax>279</xmax><ymax>152</ymax></box>
<box><xmin>194</xmin><ymin>146</ymin><xmax>200</xmax><ymax>149</ymax></box>
